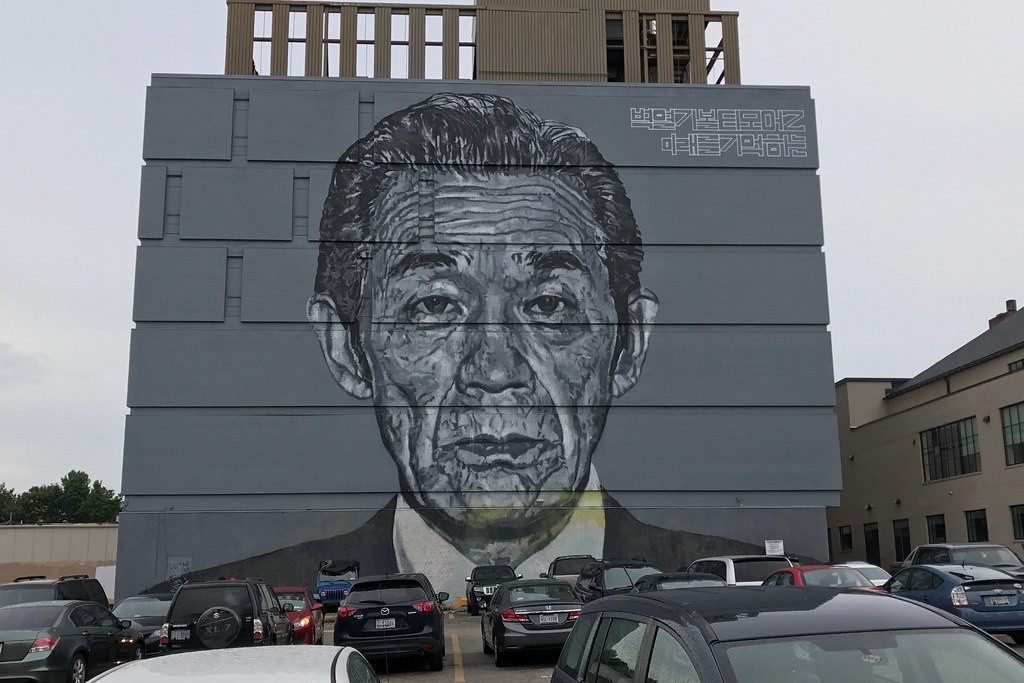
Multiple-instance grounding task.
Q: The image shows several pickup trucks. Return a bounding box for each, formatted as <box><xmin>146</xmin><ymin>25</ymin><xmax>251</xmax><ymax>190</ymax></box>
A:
<box><xmin>316</xmin><ymin>558</ymin><xmax>359</xmax><ymax>610</ymax></box>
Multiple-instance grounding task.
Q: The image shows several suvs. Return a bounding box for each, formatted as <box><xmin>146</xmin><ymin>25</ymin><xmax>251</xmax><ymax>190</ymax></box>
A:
<box><xmin>160</xmin><ymin>575</ymin><xmax>297</xmax><ymax>654</ymax></box>
<box><xmin>333</xmin><ymin>572</ymin><xmax>449</xmax><ymax>671</ymax></box>
<box><xmin>465</xmin><ymin>565</ymin><xmax>523</xmax><ymax>615</ymax></box>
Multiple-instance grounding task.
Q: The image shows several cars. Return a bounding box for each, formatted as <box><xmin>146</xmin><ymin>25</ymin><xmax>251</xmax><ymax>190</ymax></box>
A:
<box><xmin>481</xmin><ymin>576</ymin><xmax>584</xmax><ymax>666</ymax></box>
<box><xmin>539</xmin><ymin>543</ymin><xmax>1024</xmax><ymax>642</ymax></box>
<box><xmin>0</xmin><ymin>600</ymin><xmax>144</xmax><ymax>683</ymax></box>
<box><xmin>82</xmin><ymin>645</ymin><xmax>380</xmax><ymax>683</ymax></box>
<box><xmin>0</xmin><ymin>575</ymin><xmax>325</xmax><ymax>645</ymax></box>
<box><xmin>549</xmin><ymin>586</ymin><xmax>1024</xmax><ymax>683</ymax></box>
